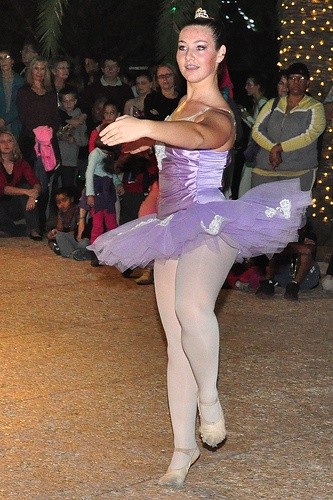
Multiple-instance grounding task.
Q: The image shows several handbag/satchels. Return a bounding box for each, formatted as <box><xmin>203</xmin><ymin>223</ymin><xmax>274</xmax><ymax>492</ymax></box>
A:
<box><xmin>244</xmin><ymin>96</ymin><xmax>279</xmax><ymax>162</ymax></box>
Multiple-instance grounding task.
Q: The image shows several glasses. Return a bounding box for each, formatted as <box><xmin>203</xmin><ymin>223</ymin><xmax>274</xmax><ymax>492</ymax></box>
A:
<box><xmin>20</xmin><ymin>49</ymin><xmax>32</xmax><ymax>54</ymax></box>
<box><xmin>156</xmin><ymin>73</ymin><xmax>173</xmax><ymax>79</ymax></box>
<box><xmin>0</xmin><ymin>55</ymin><xmax>11</xmax><ymax>60</ymax></box>
<box><xmin>288</xmin><ymin>76</ymin><xmax>307</xmax><ymax>81</ymax></box>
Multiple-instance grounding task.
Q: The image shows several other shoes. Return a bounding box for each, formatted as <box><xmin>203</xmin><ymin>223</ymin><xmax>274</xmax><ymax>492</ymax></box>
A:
<box><xmin>12</xmin><ymin>227</ymin><xmax>27</xmax><ymax>237</ymax></box>
<box><xmin>53</xmin><ymin>245</ymin><xmax>61</xmax><ymax>256</ymax></box>
<box><xmin>321</xmin><ymin>275</ymin><xmax>333</xmax><ymax>291</ymax></box>
<box><xmin>159</xmin><ymin>443</ymin><xmax>200</xmax><ymax>487</ymax></box>
<box><xmin>197</xmin><ymin>390</ymin><xmax>226</xmax><ymax>447</ymax></box>
<box><xmin>132</xmin><ymin>268</ymin><xmax>152</xmax><ymax>284</ymax></box>
<box><xmin>30</xmin><ymin>231</ymin><xmax>44</xmax><ymax>241</ymax></box>
<box><xmin>256</xmin><ymin>280</ymin><xmax>274</xmax><ymax>300</ymax></box>
<box><xmin>285</xmin><ymin>282</ymin><xmax>298</xmax><ymax>302</ymax></box>
<box><xmin>72</xmin><ymin>248</ymin><xmax>92</xmax><ymax>261</ymax></box>
<box><xmin>89</xmin><ymin>250</ymin><xmax>99</xmax><ymax>267</ymax></box>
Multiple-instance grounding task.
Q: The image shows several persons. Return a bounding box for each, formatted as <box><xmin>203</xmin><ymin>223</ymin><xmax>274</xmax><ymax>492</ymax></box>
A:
<box><xmin>87</xmin><ymin>7</ymin><xmax>313</xmax><ymax>485</ymax></box>
<box><xmin>0</xmin><ymin>40</ymin><xmax>333</xmax><ymax>301</ymax></box>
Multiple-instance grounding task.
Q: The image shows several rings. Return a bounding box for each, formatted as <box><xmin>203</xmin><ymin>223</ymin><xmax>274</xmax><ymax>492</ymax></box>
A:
<box><xmin>30</xmin><ymin>205</ymin><xmax>33</xmax><ymax>207</ymax></box>
